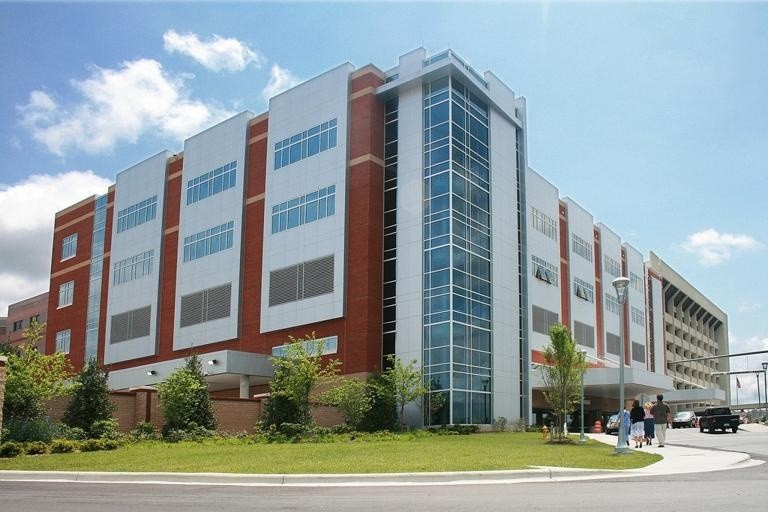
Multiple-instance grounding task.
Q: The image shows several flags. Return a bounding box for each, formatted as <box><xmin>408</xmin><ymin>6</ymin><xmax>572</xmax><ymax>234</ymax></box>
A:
<box><xmin>736</xmin><ymin>377</ymin><xmax>741</xmax><ymax>388</ymax></box>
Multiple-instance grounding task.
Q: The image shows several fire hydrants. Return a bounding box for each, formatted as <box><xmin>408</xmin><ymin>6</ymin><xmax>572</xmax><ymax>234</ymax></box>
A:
<box><xmin>541</xmin><ymin>425</ymin><xmax>549</xmax><ymax>440</ymax></box>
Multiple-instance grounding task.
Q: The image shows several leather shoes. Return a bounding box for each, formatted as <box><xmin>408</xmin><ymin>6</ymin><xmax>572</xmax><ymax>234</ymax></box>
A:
<box><xmin>626</xmin><ymin>441</ymin><xmax>665</xmax><ymax>449</ymax></box>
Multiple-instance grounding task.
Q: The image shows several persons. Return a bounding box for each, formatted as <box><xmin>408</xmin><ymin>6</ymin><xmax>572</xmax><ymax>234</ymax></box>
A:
<box><xmin>613</xmin><ymin>406</ymin><xmax>631</xmax><ymax>446</ymax></box>
<box><xmin>630</xmin><ymin>399</ymin><xmax>646</xmax><ymax>448</ymax></box>
<box><xmin>643</xmin><ymin>401</ymin><xmax>655</xmax><ymax>445</ymax></box>
<box><xmin>650</xmin><ymin>393</ymin><xmax>670</xmax><ymax>447</ymax></box>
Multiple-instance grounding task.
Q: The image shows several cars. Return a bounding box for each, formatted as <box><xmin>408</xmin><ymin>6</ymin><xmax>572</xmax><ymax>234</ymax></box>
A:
<box><xmin>605</xmin><ymin>414</ymin><xmax>630</xmax><ymax>434</ymax></box>
<box><xmin>672</xmin><ymin>410</ymin><xmax>698</xmax><ymax>428</ymax></box>
<box><xmin>695</xmin><ymin>415</ymin><xmax>702</xmax><ymax>427</ymax></box>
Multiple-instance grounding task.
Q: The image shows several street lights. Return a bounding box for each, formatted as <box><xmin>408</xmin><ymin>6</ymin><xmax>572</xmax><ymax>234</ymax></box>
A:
<box><xmin>578</xmin><ymin>348</ymin><xmax>588</xmax><ymax>445</ymax></box>
<box><xmin>611</xmin><ymin>276</ymin><xmax>632</xmax><ymax>454</ymax></box>
<box><xmin>761</xmin><ymin>361</ymin><xmax>768</xmax><ymax>425</ymax></box>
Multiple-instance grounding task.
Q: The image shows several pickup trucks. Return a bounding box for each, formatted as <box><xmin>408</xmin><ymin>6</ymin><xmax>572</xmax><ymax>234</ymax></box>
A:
<box><xmin>699</xmin><ymin>406</ymin><xmax>740</xmax><ymax>433</ymax></box>
